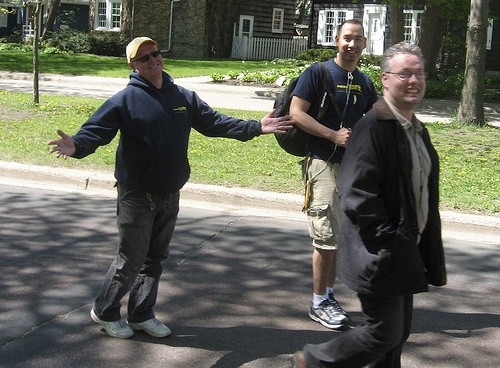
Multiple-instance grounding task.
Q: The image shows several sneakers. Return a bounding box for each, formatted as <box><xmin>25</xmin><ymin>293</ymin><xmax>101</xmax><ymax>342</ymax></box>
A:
<box><xmin>90</xmin><ymin>307</ymin><xmax>134</xmax><ymax>338</ymax></box>
<box><xmin>309</xmin><ymin>293</ymin><xmax>350</xmax><ymax>329</ymax></box>
<box><xmin>127</xmin><ymin>317</ymin><xmax>172</xmax><ymax>337</ymax></box>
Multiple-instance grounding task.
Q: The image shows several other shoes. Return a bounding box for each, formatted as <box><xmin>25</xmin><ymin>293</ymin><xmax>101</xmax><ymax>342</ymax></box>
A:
<box><xmin>294</xmin><ymin>351</ymin><xmax>305</xmax><ymax>368</ymax></box>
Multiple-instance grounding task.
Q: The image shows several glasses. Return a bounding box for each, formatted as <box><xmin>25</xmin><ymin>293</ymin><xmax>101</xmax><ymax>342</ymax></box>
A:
<box><xmin>137</xmin><ymin>50</ymin><xmax>160</xmax><ymax>62</ymax></box>
<box><xmin>386</xmin><ymin>70</ymin><xmax>427</xmax><ymax>79</ymax></box>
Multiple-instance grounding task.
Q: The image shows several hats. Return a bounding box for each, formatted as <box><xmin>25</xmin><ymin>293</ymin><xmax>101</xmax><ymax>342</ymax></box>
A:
<box><xmin>126</xmin><ymin>36</ymin><xmax>157</xmax><ymax>63</ymax></box>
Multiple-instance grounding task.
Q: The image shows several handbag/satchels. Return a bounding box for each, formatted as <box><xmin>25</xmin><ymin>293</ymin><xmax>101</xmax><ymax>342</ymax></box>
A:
<box><xmin>272</xmin><ymin>62</ymin><xmax>336</xmax><ymax>158</ymax></box>
